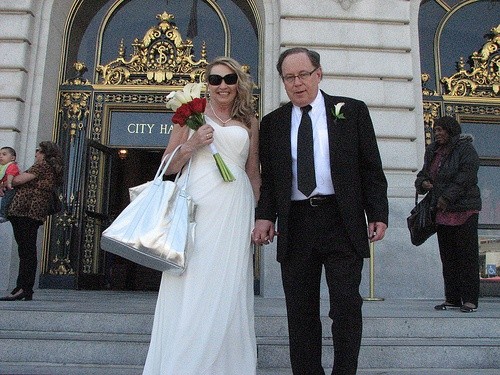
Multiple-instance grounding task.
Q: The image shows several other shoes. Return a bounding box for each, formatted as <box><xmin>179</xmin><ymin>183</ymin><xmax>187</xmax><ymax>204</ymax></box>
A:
<box><xmin>0</xmin><ymin>214</ymin><xmax>9</xmax><ymax>222</ymax></box>
<box><xmin>434</xmin><ymin>302</ymin><xmax>461</xmax><ymax>310</ymax></box>
<box><xmin>460</xmin><ymin>304</ymin><xmax>478</xmax><ymax>312</ymax></box>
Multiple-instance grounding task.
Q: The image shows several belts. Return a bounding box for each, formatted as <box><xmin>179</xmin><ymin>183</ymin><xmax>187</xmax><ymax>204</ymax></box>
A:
<box><xmin>293</xmin><ymin>196</ymin><xmax>336</xmax><ymax>207</ymax></box>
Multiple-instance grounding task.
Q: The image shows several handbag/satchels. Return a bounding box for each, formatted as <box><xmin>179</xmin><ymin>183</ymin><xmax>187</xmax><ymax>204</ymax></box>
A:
<box><xmin>407</xmin><ymin>189</ymin><xmax>438</xmax><ymax>246</ymax></box>
<box><xmin>100</xmin><ymin>146</ymin><xmax>196</xmax><ymax>276</ymax></box>
<box><xmin>48</xmin><ymin>192</ymin><xmax>62</xmax><ymax>215</ymax></box>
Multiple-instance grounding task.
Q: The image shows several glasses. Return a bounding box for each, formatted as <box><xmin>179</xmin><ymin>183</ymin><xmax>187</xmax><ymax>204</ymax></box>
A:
<box><xmin>209</xmin><ymin>73</ymin><xmax>238</xmax><ymax>86</ymax></box>
<box><xmin>35</xmin><ymin>148</ymin><xmax>41</xmax><ymax>152</ymax></box>
<box><xmin>282</xmin><ymin>67</ymin><xmax>318</xmax><ymax>83</ymax></box>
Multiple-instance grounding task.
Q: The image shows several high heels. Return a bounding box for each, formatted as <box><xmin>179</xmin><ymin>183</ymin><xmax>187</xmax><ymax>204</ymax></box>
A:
<box><xmin>0</xmin><ymin>288</ymin><xmax>32</xmax><ymax>301</ymax></box>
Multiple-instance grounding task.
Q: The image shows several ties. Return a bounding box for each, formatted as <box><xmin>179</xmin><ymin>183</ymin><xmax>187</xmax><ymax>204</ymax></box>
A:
<box><xmin>297</xmin><ymin>106</ymin><xmax>316</xmax><ymax>197</ymax></box>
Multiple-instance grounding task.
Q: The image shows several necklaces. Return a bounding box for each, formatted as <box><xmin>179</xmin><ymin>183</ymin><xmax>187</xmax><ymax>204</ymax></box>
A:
<box><xmin>209</xmin><ymin>102</ymin><xmax>232</xmax><ymax>123</ymax></box>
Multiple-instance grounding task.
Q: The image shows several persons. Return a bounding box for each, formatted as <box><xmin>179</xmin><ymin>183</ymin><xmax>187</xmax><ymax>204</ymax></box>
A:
<box><xmin>0</xmin><ymin>146</ymin><xmax>20</xmax><ymax>223</ymax></box>
<box><xmin>143</xmin><ymin>56</ymin><xmax>277</xmax><ymax>375</ymax></box>
<box><xmin>0</xmin><ymin>140</ymin><xmax>65</xmax><ymax>301</ymax></box>
<box><xmin>413</xmin><ymin>115</ymin><xmax>482</xmax><ymax>313</ymax></box>
<box><xmin>250</xmin><ymin>47</ymin><xmax>389</xmax><ymax>375</ymax></box>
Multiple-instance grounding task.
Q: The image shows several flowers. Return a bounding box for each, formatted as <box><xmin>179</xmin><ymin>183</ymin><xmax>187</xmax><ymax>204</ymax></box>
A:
<box><xmin>165</xmin><ymin>83</ymin><xmax>236</xmax><ymax>182</ymax></box>
<box><xmin>332</xmin><ymin>102</ymin><xmax>345</xmax><ymax>122</ymax></box>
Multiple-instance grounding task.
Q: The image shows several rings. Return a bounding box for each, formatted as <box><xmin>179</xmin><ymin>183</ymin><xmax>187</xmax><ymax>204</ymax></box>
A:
<box><xmin>207</xmin><ymin>135</ymin><xmax>209</xmax><ymax>138</ymax></box>
